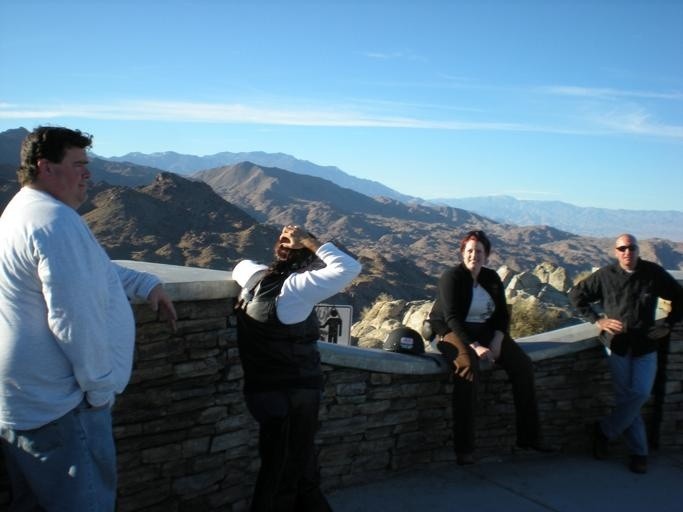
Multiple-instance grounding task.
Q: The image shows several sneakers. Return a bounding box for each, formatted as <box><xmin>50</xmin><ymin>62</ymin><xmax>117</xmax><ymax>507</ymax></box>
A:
<box><xmin>630</xmin><ymin>455</ymin><xmax>647</xmax><ymax>473</ymax></box>
<box><xmin>457</xmin><ymin>452</ymin><xmax>476</xmax><ymax>465</ymax></box>
<box><xmin>530</xmin><ymin>440</ymin><xmax>552</xmax><ymax>452</ymax></box>
<box><xmin>583</xmin><ymin>435</ymin><xmax>596</xmax><ymax>457</ymax></box>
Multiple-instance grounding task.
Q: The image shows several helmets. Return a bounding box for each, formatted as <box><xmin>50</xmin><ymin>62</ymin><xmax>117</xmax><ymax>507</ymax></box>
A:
<box><xmin>383</xmin><ymin>327</ymin><xmax>425</xmax><ymax>354</ymax></box>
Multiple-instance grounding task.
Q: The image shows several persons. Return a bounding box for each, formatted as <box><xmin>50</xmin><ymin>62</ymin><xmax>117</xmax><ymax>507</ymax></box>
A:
<box><xmin>568</xmin><ymin>232</ymin><xmax>683</xmax><ymax>473</ymax></box>
<box><xmin>0</xmin><ymin>124</ymin><xmax>180</xmax><ymax>512</ymax></box>
<box><xmin>422</xmin><ymin>230</ymin><xmax>555</xmax><ymax>465</ymax></box>
<box><xmin>232</xmin><ymin>223</ymin><xmax>363</xmax><ymax>512</ymax></box>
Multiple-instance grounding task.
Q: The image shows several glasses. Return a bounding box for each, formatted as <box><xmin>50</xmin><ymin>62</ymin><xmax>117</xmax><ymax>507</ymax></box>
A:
<box><xmin>616</xmin><ymin>246</ymin><xmax>636</xmax><ymax>251</ymax></box>
